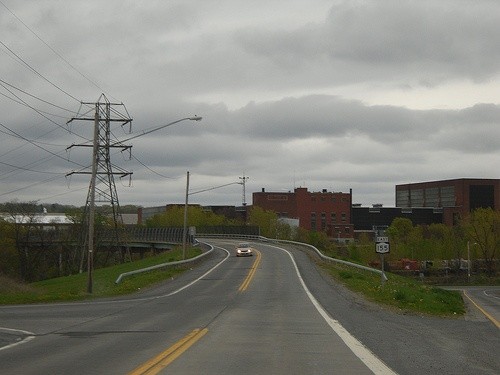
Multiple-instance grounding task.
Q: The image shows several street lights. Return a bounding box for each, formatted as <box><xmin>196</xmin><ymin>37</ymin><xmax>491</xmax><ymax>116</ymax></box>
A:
<box><xmin>87</xmin><ymin>115</ymin><xmax>203</xmax><ymax>293</ymax></box>
<box><xmin>181</xmin><ymin>170</ymin><xmax>243</xmax><ymax>261</ymax></box>
<box><xmin>467</xmin><ymin>240</ymin><xmax>477</xmax><ymax>278</ymax></box>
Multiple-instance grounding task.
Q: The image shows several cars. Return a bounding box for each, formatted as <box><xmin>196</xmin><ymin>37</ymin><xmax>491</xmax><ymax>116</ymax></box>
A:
<box><xmin>234</xmin><ymin>242</ymin><xmax>254</xmax><ymax>257</ymax></box>
<box><xmin>368</xmin><ymin>258</ymin><xmax>468</xmax><ymax>271</ymax></box>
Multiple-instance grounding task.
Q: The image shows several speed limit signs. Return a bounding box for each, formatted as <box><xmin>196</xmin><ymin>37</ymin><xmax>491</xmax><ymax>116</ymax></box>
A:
<box><xmin>375</xmin><ymin>241</ymin><xmax>390</xmax><ymax>254</ymax></box>
